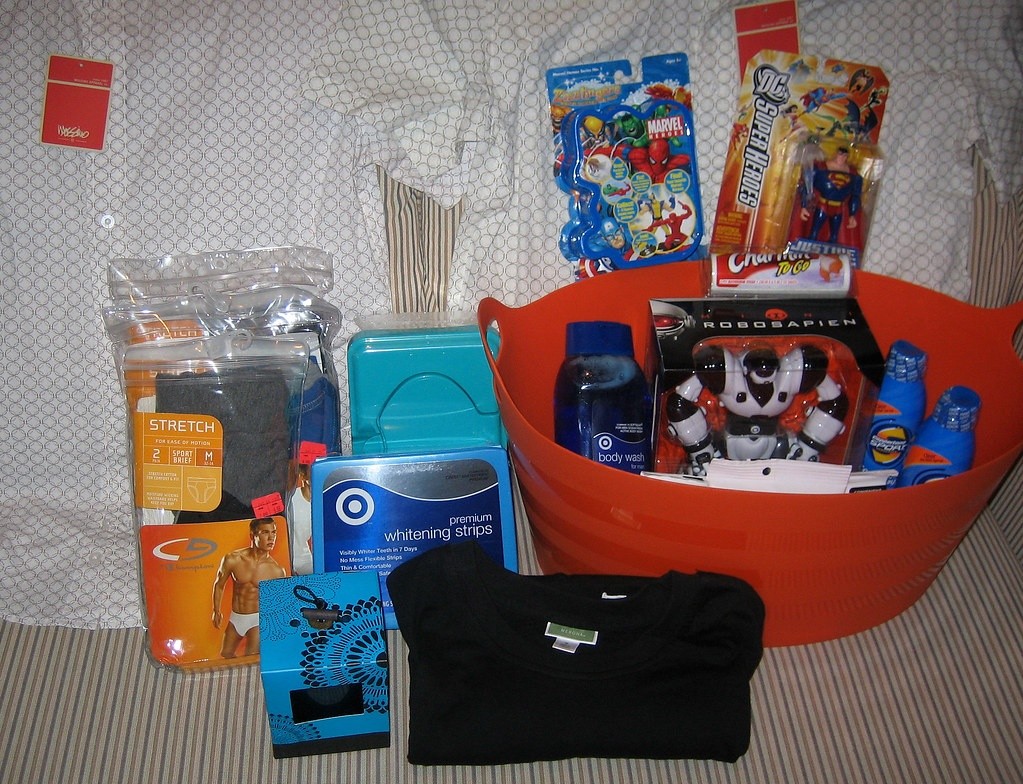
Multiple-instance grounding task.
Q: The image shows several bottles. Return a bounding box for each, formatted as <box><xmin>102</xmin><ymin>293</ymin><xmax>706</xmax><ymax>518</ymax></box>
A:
<box><xmin>863</xmin><ymin>339</ymin><xmax>926</xmax><ymax>471</ymax></box>
<box><xmin>894</xmin><ymin>384</ymin><xmax>980</xmax><ymax>488</ymax></box>
<box><xmin>552</xmin><ymin>321</ymin><xmax>651</xmax><ymax>477</ymax></box>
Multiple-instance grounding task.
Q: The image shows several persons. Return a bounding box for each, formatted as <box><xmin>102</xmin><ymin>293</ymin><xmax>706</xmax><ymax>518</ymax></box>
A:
<box><xmin>211</xmin><ymin>518</ymin><xmax>286</xmax><ymax>659</ymax></box>
<box><xmin>798</xmin><ymin>146</ymin><xmax>863</xmax><ymax>242</ymax></box>
<box><xmin>289</xmin><ymin>463</ymin><xmax>313</xmax><ymax>576</ymax></box>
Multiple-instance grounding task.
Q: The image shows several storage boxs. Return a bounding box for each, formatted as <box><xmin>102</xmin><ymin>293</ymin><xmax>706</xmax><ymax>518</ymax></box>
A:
<box><xmin>645</xmin><ymin>292</ymin><xmax>887</xmax><ymax>473</ymax></box>
<box><xmin>311</xmin><ymin>313</ymin><xmax>520</xmax><ymax>631</ymax></box>
<box><xmin>258</xmin><ymin>568</ymin><xmax>391</xmax><ymax>760</ymax></box>
